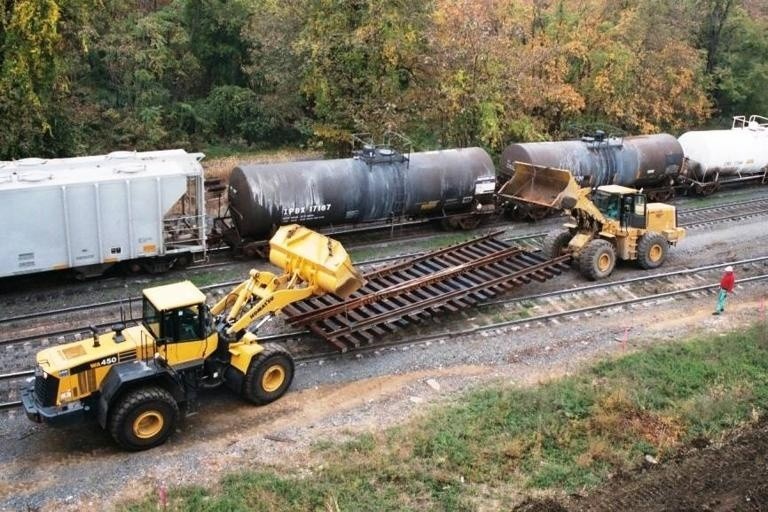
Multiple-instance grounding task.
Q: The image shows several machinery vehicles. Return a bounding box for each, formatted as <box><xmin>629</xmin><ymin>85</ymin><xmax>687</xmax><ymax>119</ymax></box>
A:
<box><xmin>20</xmin><ymin>224</ymin><xmax>368</xmax><ymax>454</ymax></box>
<box><xmin>498</xmin><ymin>161</ymin><xmax>686</xmax><ymax>279</ymax></box>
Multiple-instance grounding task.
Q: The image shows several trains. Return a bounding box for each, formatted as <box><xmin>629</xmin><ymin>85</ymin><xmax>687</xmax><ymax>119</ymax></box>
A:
<box><xmin>0</xmin><ymin>113</ymin><xmax>765</xmax><ymax>287</ymax></box>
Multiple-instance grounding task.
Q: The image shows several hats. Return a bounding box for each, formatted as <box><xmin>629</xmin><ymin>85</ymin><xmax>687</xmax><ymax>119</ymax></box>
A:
<box><xmin>724</xmin><ymin>266</ymin><xmax>733</xmax><ymax>272</ymax></box>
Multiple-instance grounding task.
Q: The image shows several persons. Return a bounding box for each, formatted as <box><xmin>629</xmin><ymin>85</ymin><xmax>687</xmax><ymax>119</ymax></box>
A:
<box><xmin>607</xmin><ymin>200</ymin><xmax>618</xmax><ymax>217</ymax></box>
<box><xmin>176</xmin><ymin>311</ymin><xmax>198</xmax><ymax>339</ymax></box>
<box><xmin>712</xmin><ymin>266</ymin><xmax>734</xmax><ymax>315</ymax></box>
